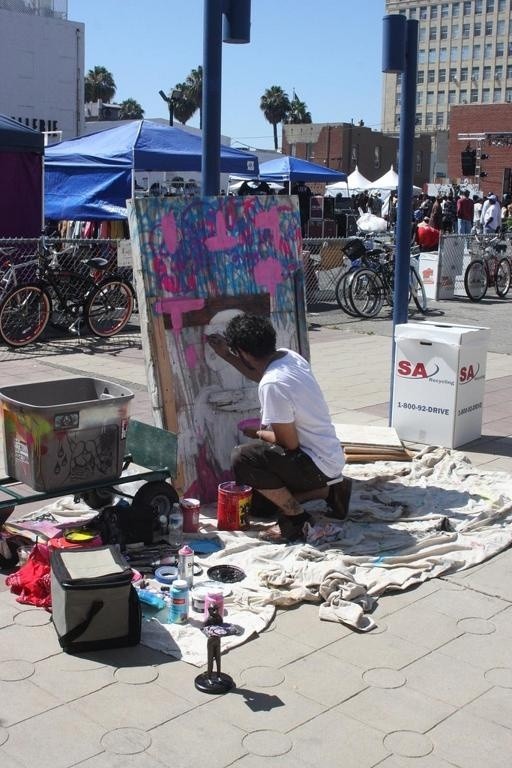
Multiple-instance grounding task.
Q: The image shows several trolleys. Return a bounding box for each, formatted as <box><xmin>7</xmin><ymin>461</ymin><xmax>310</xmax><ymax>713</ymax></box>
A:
<box><xmin>0</xmin><ymin>418</ymin><xmax>180</xmax><ymax>570</ymax></box>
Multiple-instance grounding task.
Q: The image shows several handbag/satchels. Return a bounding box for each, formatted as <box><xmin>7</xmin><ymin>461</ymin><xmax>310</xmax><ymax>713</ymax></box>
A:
<box><xmin>51</xmin><ymin>546</ymin><xmax>142</xmax><ymax>653</ymax></box>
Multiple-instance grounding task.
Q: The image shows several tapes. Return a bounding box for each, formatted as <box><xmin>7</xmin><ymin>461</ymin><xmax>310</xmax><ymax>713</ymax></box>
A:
<box><xmin>154</xmin><ymin>565</ymin><xmax>179</xmax><ymax>584</ymax></box>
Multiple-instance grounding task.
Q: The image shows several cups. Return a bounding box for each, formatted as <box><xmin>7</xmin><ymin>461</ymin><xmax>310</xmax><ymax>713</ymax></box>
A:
<box><xmin>17</xmin><ymin>545</ymin><xmax>37</xmax><ymax>569</ymax></box>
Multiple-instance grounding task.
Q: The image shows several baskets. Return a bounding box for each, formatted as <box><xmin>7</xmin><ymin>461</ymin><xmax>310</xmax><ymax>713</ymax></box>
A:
<box><xmin>342</xmin><ymin>239</ymin><xmax>365</xmax><ymax>261</ymax></box>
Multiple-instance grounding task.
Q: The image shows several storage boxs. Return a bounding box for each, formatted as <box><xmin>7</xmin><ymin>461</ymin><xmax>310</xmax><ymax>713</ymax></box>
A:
<box><xmin>0</xmin><ymin>378</ymin><xmax>135</xmax><ymax>492</ymax></box>
<box><xmin>391</xmin><ymin>320</ymin><xmax>491</xmax><ymax>449</ymax></box>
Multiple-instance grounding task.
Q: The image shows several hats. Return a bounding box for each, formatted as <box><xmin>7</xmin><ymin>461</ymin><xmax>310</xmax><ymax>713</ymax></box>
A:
<box><xmin>372</xmin><ymin>190</ymin><xmax>377</xmax><ymax>197</ymax></box>
<box><xmin>487</xmin><ymin>195</ymin><xmax>497</xmax><ymax>202</ymax></box>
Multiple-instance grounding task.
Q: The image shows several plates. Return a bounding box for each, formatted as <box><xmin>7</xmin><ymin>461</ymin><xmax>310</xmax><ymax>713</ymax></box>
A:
<box><xmin>237</xmin><ymin>417</ymin><xmax>268</xmax><ymax>432</ymax></box>
<box><xmin>63</xmin><ymin>529</ymin><xmax>100</xmax><ymax>542</ymax></box>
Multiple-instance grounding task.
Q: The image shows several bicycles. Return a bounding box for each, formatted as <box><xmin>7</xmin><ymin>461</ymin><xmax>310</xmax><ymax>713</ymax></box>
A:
<box><xmin>334</xmin><ymin>231</ymin><xmax>429</xmax><ymax>317</ymax></box>
<box><xmin>464</xmin><ymin>232</ymin><xmax>512</xmax><ymax>301</ymax></box>
<box><xmin>0</xmin><ymin>225</ymin><xmax>136</xmax><ymax>346</ymax></box>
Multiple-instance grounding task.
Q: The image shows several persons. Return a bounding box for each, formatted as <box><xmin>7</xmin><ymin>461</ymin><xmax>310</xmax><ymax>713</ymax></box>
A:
<box><xmin>237</xmin><ymin>181</ymin><xmax>510</xmax><ymax>251</ymax></box>
<box><xmin>209</xmin><ymin>315</ymin><xmax>352</xmax><ymax>542</ymax></box>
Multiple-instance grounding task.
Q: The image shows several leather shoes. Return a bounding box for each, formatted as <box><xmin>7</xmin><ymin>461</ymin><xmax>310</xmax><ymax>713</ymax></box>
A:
<box><xmin>258</xmin><ymin>511</ymin><xmax>316</xmax><ymax>544</ymax></box>
<box><xmin>324</xmin><ymin>478</ymin><xmax>352</xmax><ymax>520</ymax></box>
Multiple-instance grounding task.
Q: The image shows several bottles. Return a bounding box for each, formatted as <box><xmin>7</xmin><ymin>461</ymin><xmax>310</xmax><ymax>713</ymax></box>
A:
<box><xmin>177</xmin><ymin>545</ymin><xmax>194</xmax><ymax>589</ymax></box>
<box><xmin>168</xmin><ymin>579</ymin><xmax>191</xmax><ymax>624</ymax></box>
<box><xmin>180</xmin><ymin>497</ymin><xmax>201</xmax><ymax>534</ymax></box>
<box><xmin>191</xmin><ymin>588</ymin><xmax>204</xmax><ymax>613</ymax></box>
<box><xmin>203</xmin><ymin>588</ymin><xmax>225</xmax><ymax>620</ymax></box>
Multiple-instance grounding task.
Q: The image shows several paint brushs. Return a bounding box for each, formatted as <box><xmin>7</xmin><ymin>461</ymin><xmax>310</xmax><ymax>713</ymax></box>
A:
<box><xmin>199</xmin><ymin>332</ymin><xmax>227</xmax><ymax>345</ymax></box>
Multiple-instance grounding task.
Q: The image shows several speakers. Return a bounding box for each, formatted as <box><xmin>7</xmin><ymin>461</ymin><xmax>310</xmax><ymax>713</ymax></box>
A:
<box><xmin>335</xmin><ymin>213</ymin><xmax>359</xmax><ymax>239</ymax></box>
<box><xmin>309</xmin><ymin>195</ymin><xmax>335</xmax><ymax>220</ymax></box>
<box><xmin>461</xmin><ymin>150</ymin><xmax>475</xmax><ymax>176</ymax></box>
<box><xmin>306</xmin><ymin>220</ymin><xmax>336</xmax><ymax>239</ymax></box>
